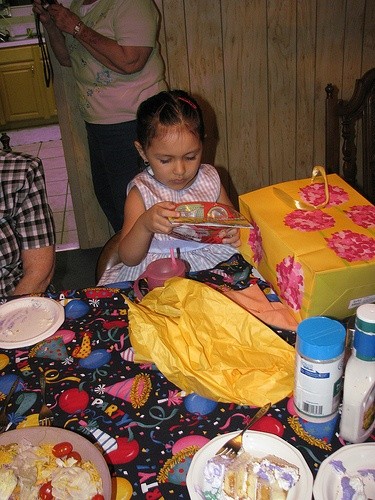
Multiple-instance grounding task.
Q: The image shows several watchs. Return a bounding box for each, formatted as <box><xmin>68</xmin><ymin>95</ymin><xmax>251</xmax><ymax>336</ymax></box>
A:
<box><xmin>73</xmin><ymin>21</ymin><xmax>83</xmax><ymax>38</ymax></box>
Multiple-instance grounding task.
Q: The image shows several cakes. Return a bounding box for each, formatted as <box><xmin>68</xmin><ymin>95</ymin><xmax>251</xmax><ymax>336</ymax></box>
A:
<box><xmin>200</xmin><ymin>454</ymin><xmax>301</xmax><ymax>500</ymax></box>
<box><xmin>0</xmin><ymin>438</ymin><xmax>97</xmax><ymax>500</ymax></box>
<box><xmin>329</xmin><ymin>460</ymin><xmax>375</xmax><ymax>500</ymax></box>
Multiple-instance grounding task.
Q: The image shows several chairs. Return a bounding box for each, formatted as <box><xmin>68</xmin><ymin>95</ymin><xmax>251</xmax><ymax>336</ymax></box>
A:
<box><xmin>324</xmin><ymin>65</ymin><xmax>375</xmax><ymax>205</ymax></box>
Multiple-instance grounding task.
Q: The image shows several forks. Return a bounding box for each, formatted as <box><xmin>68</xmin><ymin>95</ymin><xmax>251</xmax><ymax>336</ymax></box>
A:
<box><xmin>215</xmin><ymin>403</ymin><xmax>271</xmax><ymax>459</ymax></box>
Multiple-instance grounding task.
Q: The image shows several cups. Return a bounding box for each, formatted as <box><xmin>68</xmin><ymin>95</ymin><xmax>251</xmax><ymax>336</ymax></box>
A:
<box><xmin>132</xmin><ymin>258</ymin><xmax>186</xmax><ymax>302</ymax></box>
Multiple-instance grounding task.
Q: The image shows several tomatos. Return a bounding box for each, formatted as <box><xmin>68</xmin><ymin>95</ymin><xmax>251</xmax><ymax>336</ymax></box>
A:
<box><xmin>52</xmin><ymin>442</ymin><xmax>73</xmax><ymax>458</ymax></box>
<box><xmin>91</xmin><ymin>493</ymin><xmax>104</xmax><ymax>500</ymax></box>
<box><xmin>66</xmin><ymin>451</ymin><xmax>82</xmax><ymax>462</ymax></box>
<box><xmin>39</xmin><ymin>480</ymin><xmax>53</xmax><ymax>500</ymax></box>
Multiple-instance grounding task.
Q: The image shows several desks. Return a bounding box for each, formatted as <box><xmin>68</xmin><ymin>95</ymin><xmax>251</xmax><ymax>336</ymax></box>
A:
<box><xmin>0</xmin><ymin>268</ymin><xmax>351</xmax><ymax>500</ymax></box>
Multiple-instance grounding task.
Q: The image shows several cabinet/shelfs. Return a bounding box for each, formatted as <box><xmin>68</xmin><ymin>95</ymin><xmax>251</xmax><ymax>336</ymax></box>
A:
<box><xmin>0</xmin><ymin>46</ymin><xmax>58</xmax><ymax>126</ymax></box>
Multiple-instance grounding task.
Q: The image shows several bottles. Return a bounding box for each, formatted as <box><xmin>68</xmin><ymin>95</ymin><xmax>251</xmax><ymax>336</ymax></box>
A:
<box><xmin>291</xmin><ymin>316</ymin><xmax>347</xmax><ymax>423</ymax></box>
<box><xmin>340</xmin><ymin>302</ymin><xmax>375</xmax><ymax>445</ymax></box>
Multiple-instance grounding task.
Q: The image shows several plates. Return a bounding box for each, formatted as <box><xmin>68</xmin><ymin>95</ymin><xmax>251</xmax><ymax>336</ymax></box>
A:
<box><xmin>0</xmin><ymin>296</ymin><xmax>66</xmax><ymax>349</ymax></box>
<box><xmin>185</xmin><ymin>429</ymin><xmax>314</xmax><ymax>500</ymax></box>
<box><xmin>312</xmin><ymin>442</ymin><xmax>375</xmax><ymax>500</ymax></box>
<box><xmin>0</xmin><ymin>425</ymin><xmax>113</xmax><ymax>500</ymax></box>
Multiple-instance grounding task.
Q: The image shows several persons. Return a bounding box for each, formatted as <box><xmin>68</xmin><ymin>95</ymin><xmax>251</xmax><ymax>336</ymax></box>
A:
<box><xmin>0</xmin><ymin>146</ymin><xmax>56</xmax><ymax>303</ymax></box>
<box><xmin>96</xmin><ymin>89</ymin><xmax>265</xmax><ymax>287</ymax></box>
<box><xmin>32</xmin><ymin>0</ymin><xmax>169</xmax><ymax>234</ymax></box>
